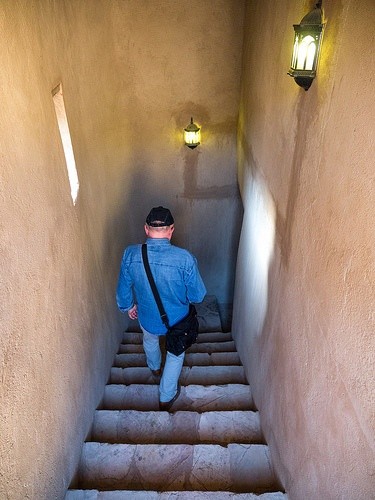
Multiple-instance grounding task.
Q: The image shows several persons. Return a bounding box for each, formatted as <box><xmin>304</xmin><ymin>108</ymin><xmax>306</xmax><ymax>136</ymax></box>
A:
<box><xmin>115</xmin><ymin>206</ymin><xmax>207</xmax><ymax>413</ymax></box>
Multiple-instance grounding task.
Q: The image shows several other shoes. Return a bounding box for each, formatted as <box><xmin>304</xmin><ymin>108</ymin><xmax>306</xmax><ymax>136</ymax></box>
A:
<box><xmin>152</xmin><ymin>365</ymin><xmax>162</xmax><ymax>378</ymax></box>
<box><xmin>159</xmin><ymin>386</ymin><xmax>180</xmax><ymax>412</ymax></box>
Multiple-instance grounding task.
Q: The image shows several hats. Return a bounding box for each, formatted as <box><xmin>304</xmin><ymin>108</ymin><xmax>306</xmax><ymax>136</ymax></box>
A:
<box><xmin>146</xmin><ymin>206</ymin><xmax>174</xmax><ymax>227</ymax></box>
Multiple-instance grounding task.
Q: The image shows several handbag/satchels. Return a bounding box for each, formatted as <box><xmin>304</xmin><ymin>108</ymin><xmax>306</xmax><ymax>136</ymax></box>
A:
<box><xmin>165</xmin><ymin>305</ymin><xmax>200</xmax><ymax>356</ymax></box>
